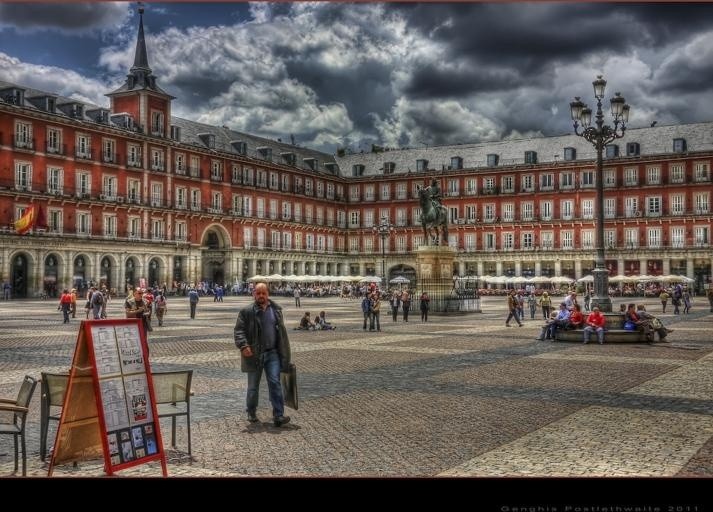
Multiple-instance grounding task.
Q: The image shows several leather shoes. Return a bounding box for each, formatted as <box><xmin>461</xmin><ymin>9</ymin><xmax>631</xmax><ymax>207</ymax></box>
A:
<box><xmin>248</xmin><ymin>412</ymin><xmax>258</xmax><ymax>422</ymax></box>
<box><xmin>274</xmin><ymin>415</ymin><xmax>290</xmax><ymax>426</ymax></box>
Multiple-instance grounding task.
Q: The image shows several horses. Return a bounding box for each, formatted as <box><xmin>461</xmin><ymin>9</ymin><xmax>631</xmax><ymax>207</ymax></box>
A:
<box><xmin>414</xmin><ymin>182</ymin><xmax>448</xmax><ymax>247</ymax></box>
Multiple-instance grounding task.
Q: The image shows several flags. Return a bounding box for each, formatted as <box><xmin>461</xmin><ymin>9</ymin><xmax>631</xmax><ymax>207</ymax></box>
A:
<box><xmin>8</xmin><ymin>200</ymin><xmax>37</xmax><ymax>233</ymax></box>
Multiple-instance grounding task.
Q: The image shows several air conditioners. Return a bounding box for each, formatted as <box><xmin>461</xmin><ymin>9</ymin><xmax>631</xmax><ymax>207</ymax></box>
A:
<box><xmin>634</xmin><ymin>210</ymin><xmax>643</xmax><ymax>217</ymax></box>
<box><xmin>51</xmin><ymin>189</ymin><xmax>242</xmax><ymax>217</ymax></box>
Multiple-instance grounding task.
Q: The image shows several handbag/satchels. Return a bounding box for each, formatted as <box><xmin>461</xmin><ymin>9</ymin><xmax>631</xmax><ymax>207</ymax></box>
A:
<box><xmin>280</xmin><ymin>363</ymin><xmax>298</xmax><ymax>410</ymax></box>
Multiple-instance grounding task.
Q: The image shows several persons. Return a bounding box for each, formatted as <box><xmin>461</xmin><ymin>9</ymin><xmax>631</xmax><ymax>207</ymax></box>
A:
<box><xmin>48</xmin><ymin>269</ymin><xmax>713</xmax><ymax>367</ymax></box>
<box><xmin>420</xmin><ymin>174</ymin><xmax>445</xmax><ymax>225</ymax></box>
<box><xmin>2</xmin><ymin>279</ymin><xmax>12</xmax><ymax>302</ymax></box>
<box><xmin>233</xmin><ymin>282</ymin><xmax>291</xmax><ymax>426</ymax></box>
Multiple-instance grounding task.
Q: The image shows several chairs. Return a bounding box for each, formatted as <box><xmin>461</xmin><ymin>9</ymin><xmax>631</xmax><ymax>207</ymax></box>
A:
<box><xmin>37</xmin><ymin>371</ymin><xmax>76</xmax><ymax>467</ymax></box>
<box><xmin>0</xmin><ymin>374</ymin><xmax>37</xmax><ymax>475</ymax></box>
<box><xmin>150</xmin><ymin>369</ymin><xmax>193</xmax><ymax>455</ymax></box>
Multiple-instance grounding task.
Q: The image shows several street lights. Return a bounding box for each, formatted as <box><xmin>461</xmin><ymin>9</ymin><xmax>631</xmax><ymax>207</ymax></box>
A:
<box><xmin>568</xmin><ymin>75</ymin><xmax>629</xmax><ymax>315</ymax></box>
<box><xmin>372</xmin><ymin>217</ymin><xmax>395</xmax><ymax>291</ymax></box>
